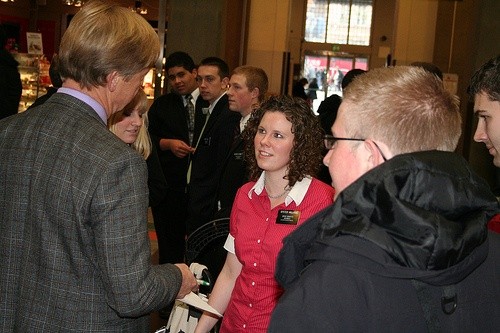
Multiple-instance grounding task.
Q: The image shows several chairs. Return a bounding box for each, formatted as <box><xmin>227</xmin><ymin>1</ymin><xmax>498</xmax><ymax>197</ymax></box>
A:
<box><xmin>184</xmin><ymin>218</ymin><xmax>230</xmax><ymax>293</ymax></box>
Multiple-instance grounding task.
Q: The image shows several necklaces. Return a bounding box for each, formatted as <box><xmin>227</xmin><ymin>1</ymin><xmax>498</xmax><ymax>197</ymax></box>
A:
<box><xmin>265</xmin><ymin>187</ymin><xmax>290</xmax><ymax>199</ymax></box>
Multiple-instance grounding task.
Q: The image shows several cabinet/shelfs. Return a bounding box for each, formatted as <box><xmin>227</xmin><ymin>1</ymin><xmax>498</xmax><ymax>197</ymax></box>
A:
<box><xmin>15</xmin><ymin>52</ymin><xmax>53</xmax><ymax>113</ymax></box>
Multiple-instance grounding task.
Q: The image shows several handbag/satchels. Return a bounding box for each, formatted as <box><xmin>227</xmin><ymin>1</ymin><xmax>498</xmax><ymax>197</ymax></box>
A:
<box><xmin>163</xmin><ymin>263</ymin><xmax>218</xmax><ymax>333</ymax></box>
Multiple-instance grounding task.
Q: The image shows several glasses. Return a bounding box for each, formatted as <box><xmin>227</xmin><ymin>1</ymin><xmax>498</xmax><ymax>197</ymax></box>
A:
<box><xmin>323</xmin><ymin>134</ymin><xmax>387</xmax><ymax>163</ymax></box>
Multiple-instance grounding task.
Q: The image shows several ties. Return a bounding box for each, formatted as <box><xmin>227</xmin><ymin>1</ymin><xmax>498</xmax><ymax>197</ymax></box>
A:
<box><xmin>184</xmin><ymin>94</ymin><xmax>194</xmax><ymax>147</ymax></box>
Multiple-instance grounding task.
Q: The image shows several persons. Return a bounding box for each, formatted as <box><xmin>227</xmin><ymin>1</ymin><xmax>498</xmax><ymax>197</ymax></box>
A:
<box><xmin>409</xmin><ymin>56</ymin><xmax>500</xmax><ymax>240</ymax></box>
<box><xmin>106</xmin><ymin>88</ymin><xmax>153</xmax><ymax>161</ymax></box>
<box><xmin>0</xmin><ymin>19</ymin><xmax>22</xmax><ymax>121</ymax></box>
<box><xmin>25</xmin><ymin>46</ymin><xmax>65</xmax><ymax>111</ymax></box>
<box><xmin>148</xmin><ymin>51</ymin><xmax>202</xmax><ymax>319</ymax></box>
<box><xmin>0</xmin><ymin>1</ymin><xmax>200</xmax><ymax>333</ymax></box>
<box><xmin>192</xmin><ymin>94</ymin><xmax>336</xmax><ymax>333</ymax></box>
<box><xmin>291</xmin><ymin>69</ymin><xmax>369</xmax><ymax>188</ymax></box>
<box><xmin>211</xmin><ymin>66</ymin><xmax>267</xmax><ymax>333</ymax></box>
<box><xmin>268</xmin><ymin>66</ymin><xmax>500</xmax><ymax>333</ymax></box>
<box><xmin>185</xmin><ymin>57</ymin><xmax>241</xmax><ymax>333</ymax></box>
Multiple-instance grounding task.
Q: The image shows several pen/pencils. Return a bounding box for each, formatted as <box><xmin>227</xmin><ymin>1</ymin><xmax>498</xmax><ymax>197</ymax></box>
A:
<box><xmin>197</xmin><ymin>280</ymin><xmax>210</xmax><ymax>286</ymax></box>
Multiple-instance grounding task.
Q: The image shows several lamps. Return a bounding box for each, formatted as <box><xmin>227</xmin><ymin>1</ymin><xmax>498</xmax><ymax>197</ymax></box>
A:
<box><xmin>132</xmin><ymin>1</ymin><xmax>147</xmax><ymax>15</ymax></box>
<box><xmin>65</xmin><ymin>0</ymin><xmax>82</xmax><ymax>7</ymax></box>
